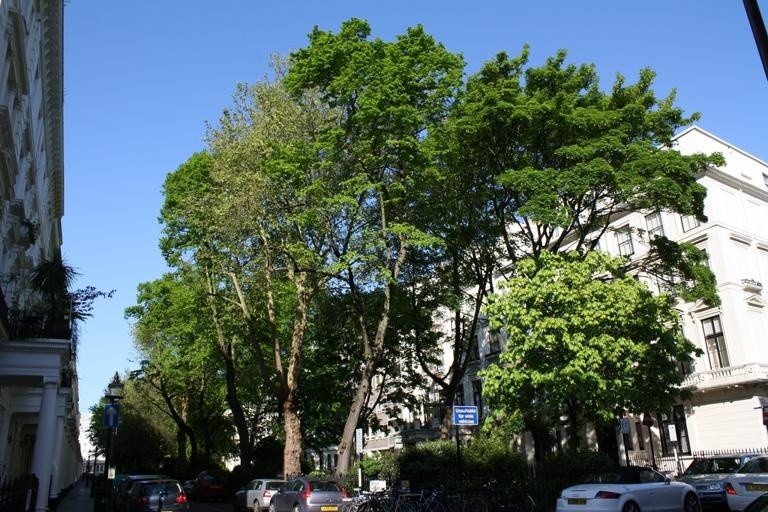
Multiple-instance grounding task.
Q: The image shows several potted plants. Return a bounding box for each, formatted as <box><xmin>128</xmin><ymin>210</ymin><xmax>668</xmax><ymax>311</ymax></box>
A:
<box><xmin>14</xmin><ymin>251</ymin><xmax>85</xmax><ymax>338</ymax></box>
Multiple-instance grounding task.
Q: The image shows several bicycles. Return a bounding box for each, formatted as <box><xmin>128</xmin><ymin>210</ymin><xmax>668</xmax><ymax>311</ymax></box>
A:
<box><xmin>345</xmin><ymin>473</ymin><xmax>537</xmax><ymax>512</ymax></box>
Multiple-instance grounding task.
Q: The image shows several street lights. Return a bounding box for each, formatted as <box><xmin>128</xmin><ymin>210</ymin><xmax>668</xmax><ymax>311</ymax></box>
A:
<box><xmin>86</xmin><ymin>370</ymin><xmax>124</xmax><ymax>512</ymax></box>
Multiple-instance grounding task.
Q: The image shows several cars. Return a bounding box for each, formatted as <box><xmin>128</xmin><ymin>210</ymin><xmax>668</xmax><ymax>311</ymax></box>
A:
<box><xmin>93</xmin><ymin>473</ymin><xmax>192</xmax><ymax>512</ymax></box>
<box><xmin>555</xmin><ymin>466</ymin><xmax>703</xmax><ymax>512</ymax></box>
<box><xmin>234</xmin><ymin>477</ymin><xmax>350</xmax><ymax>512</ymax></box>
<box><xmin>184</xmin><ymin>470</ymin><xmax>229</xmax><ymax>502</ymax></box>
<box><xmin>677</xmin><ymin>452</ymin><xmax>768</xmax><ymax>512</ymax></box>
